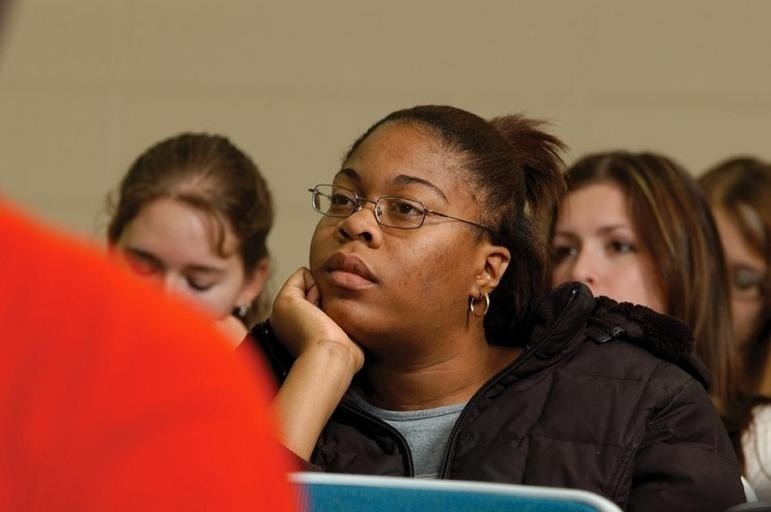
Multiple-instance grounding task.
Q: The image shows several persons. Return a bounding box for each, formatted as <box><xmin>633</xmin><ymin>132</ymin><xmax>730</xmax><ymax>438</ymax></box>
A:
<box><xmin>543</xmin><ymin>152</ymin><xmax>760</xmax><ymax>509</ymax></box>
<box><xmin>688</xmin><ymin>154</ymin><xmax>767</xmax><ymax>511</ymax></box>
<box><xmin>108</xmin><ymin>132</ymin><xmax>277</xmax><ymax>402</ymax></box>
<box><xmin>235</xmin><ymin>103</ymin><xmax>755</xmax><ymax>509</ymax></box>
<box><xmin>2</xmin><ymin>1</ymin><xmax>308</xmax><ymax>511</ymax></box>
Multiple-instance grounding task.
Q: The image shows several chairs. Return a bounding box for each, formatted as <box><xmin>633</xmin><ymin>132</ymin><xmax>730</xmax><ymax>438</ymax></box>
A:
<box><xmin>287</xmin><ymin>471</ymin><xmax>622</xmax><ymax>511</ymax></box>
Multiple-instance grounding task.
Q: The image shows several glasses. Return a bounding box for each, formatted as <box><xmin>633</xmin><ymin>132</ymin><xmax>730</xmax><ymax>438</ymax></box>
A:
<box><xmin>307</xmin><ymin>182</ymin><xmax>510</xmax><ymax>244</ymax></box>
